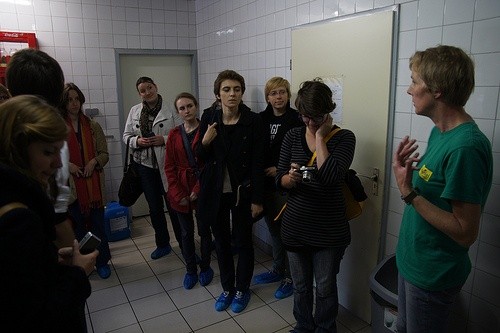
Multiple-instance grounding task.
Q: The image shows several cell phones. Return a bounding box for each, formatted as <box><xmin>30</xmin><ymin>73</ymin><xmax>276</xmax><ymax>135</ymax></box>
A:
<box><xmin>78</xmin><ymin>231</ymin><xmax>102</xmax><ymax>255</ymax></box>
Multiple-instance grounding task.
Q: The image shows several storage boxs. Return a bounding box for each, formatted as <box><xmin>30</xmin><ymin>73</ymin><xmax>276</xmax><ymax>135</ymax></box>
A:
<box><xmin>102</xmin><ymin>203</ymin><xmax>131</xmax><ymax>242</ymax></box>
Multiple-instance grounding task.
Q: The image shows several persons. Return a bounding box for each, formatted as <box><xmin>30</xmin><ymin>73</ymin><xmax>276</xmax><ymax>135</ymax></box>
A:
<box><xmin>274</xmin><ymin>82</ymin><xmax>357</xmax><ymax>333</ymax></box>
<box><xmin>254</xmin><ymin>77</ymin><xmax>307</xmax><ymax>299</ymax></box>
<box><xmin>56</xmin><ymin>83</ymin><xmax>110</xmax><ymax>277</ymax></box>
<box><xmin>118</xmin><ymin>77</ymin><xmax>185</xmax><ymax>259</ymax></box>
<box><xmin>210</xmin><ymin>207</ymin><xmax>239</xmax><ymax>257</ymax></box>
<box><xmin>164</xmin><ymin>92</ymin><xmax>214</xmax><ymax>290</ymax></box>
<box><xmin>0</xmin><ymin>91</ymin><xmax>101</xmax><ymax>333</ymax></box>
<box><xmin>6</xmin><ymin>50</ymin><xmax>77</xmax><ymax>248</ymax></box>
<box><xmin>394</xmin><ymin>44</ymin><xmax>493</xmax><ymax>333</ymax></box>
<box><xmin>191</xmin><ymin>70</ymin><xmax>264</xmax><ymax>313</ymax></box>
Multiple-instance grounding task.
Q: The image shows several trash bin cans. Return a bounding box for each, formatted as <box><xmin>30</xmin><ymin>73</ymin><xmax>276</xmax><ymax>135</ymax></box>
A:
<box><xmin>369</xmin><ymin>254</ymin><xmax>398</xmax><ymax>333</ymax></box>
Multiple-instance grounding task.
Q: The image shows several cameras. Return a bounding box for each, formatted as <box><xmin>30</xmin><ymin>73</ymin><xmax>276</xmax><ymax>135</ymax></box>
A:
<box><xmin>293</xmin><ymin>166</ymin><xmax>316</xmax><ymax>183</ymax></box>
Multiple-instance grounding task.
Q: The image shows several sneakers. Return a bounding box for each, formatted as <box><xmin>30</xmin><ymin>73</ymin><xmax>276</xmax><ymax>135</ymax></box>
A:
<box><xmin>199</xmin><ymin>268</ymin><xmax>214</xmax><ymax>286</ymax></box>
<box><xmin>254</xmin><ymin>270</ymin><xmax>285</xmax><ymax>284</ymax></box>
<box><xmin>215</xmin><ymin>287</ymin><xmax>236</xmax><ymax>312</ymax></box>
<box><xmin>275</xmin><ymin>277</ymin><xmax>294</xmax><ymax>299</ymax></box>
<box><xmin>97</xmin><ymin>264</ymin><xmax>111</xmax><ymax>278</ymax></box>
<box><xmin>194</xmin><ymin>253</ymin><xmax>201</xmax><ymax>264</ymax></box>
<box><xmin>183</xmin><ymin>271</ymin><xmax>198</xmax><ymax>289</ymax></box>
<box><xmin>231</xmin><ymin>289</ymin><xmax>251</xmax><ymax>313</ymax></box>
<box><xmin>151</xmin><ymin>244</ymin><xmax>172</xmax><ymax>259</ymax></box>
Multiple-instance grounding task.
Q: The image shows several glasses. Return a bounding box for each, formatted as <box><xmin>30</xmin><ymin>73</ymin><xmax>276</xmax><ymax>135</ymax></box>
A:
<box><xmin>298</xmin><ymin>112</ymin><xmax>326</xmax><ymax>122</ymax></box>
<box><xmin>269</xmin><ymin>90</ymin><xmax>287</xmax><ymax>96</ymax></box>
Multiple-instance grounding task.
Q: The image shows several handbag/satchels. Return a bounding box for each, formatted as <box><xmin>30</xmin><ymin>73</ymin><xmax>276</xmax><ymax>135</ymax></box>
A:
<box><xmin>341</xmin><ymin>169</ymin><xmax>367</xmax><ymax>219</ymax></box>
<box><xmin>119</xmin><ymin>165</ymin><xmax>140</xmax><ymax>206</ymax></box>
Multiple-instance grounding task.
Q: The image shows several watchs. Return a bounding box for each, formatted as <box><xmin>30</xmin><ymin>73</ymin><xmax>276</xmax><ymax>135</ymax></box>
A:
<box><xmin>401</xmin><ymin>189</ymin><xmax>418</xmax><ymax>205</ymax></box>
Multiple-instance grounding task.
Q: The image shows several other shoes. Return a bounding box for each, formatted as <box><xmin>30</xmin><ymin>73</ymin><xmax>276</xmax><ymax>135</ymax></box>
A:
<box><xmin>289</xmin><ymin>330</ymin><xmax>298</xmax><ymax>333</ymax></box>
